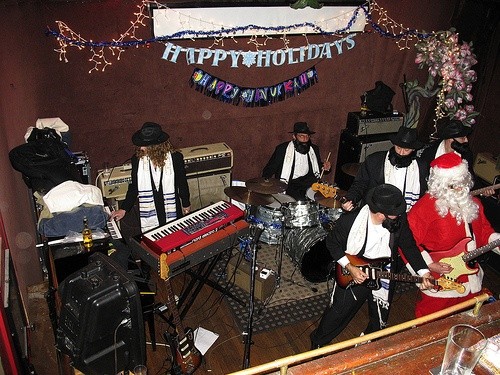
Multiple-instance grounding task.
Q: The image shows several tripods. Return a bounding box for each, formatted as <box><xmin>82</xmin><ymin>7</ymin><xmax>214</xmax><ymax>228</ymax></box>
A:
<box><xmin>256</xmin><ymin>204</ymin><xmax>318</xmax><ymax>316</ymax></box>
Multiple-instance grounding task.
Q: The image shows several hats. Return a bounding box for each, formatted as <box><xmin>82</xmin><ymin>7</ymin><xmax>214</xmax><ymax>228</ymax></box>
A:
<box><xmin>430</xmin><ymin>152</ymin><xmax>468</xmax><ymax>178</ymax></box>
<box><xmin>436</xmin><ymin>120</ymin><xmax>471</xmax><ymax>138</ymax></box>
<box><xmin>366</xmin><ymin>184</ymin><xmax>406</xmax><ymax>215</ymax></box>
<box><xmin>132</xmin><ymin>122</ymin><xmax>170</xmax><ymax>146</ymax></box>
<box><xmin>288</xmin><ymin>123</ymin><xmax>315</xmax><ymax>134</ymax></box>
<box><xmin>388</xmin><ymin>126</ymin><xmax>422</xmax><ymax>149</ymax></box>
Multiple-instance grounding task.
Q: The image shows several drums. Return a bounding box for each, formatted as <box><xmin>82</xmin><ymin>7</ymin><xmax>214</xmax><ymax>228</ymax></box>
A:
<box><xmin>284</xmin><ymin>225</ymin><xmax>336</xmax><ymax>284</ymax></box>
<box><xmin>243</xmin><ymin>192</ymin><xmax>298</xmax><ymax>245</ymax></box>
<box><xmin>279</xmin><ymin>201</ymin><xmax>320</xmax><ymax>229</ymax></box>
<box><xmin>305</xmin><ymin>187</ymin><xmax>343</xmax><ymax>222</ymax></box>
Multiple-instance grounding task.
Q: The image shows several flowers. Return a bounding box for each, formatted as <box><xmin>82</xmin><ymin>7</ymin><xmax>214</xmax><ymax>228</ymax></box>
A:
<box><xmin>414</xmin><ymin>26</ymin><xmax>479</xmax><ymax>128</ymax></box>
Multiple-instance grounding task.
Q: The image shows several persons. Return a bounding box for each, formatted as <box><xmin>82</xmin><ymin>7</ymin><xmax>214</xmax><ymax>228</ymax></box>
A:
<box><xmin>420</xmin><ymin>120</ymin><xmax>496</xmax><ymax>198</ymax></box>
<box><xmin>108</xmin><ymin>121</ymin><xmax>191</xmax><ymax>233</ymax></box>
<box><xmin>340</xmin><ymin>125</ymin><xmax>429</xmax><ymax>213</ymax></box>
<box><xmin>397</xmin><ymin>151</ymin><xmax>500</xmax><ymax>318</ymax></box>
<box><xmin>262</xmin><ymin>122</ymin><xmax>331</xmax><ymax>201</ymax></box>
<box><xmin>309</xmin><ymin>184</ymin><xmax>435</xmax><ymax>350</ymax></box>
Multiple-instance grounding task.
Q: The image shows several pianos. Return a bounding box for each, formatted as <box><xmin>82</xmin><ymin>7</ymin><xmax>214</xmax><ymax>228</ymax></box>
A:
<box><xmin>31</xmin><ymin>184</ymin><xmax>252</xmax><ymax>332</ymax></box>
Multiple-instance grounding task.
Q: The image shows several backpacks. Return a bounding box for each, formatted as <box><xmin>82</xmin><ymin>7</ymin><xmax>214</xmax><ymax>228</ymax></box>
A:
<box><xmin>8</xmin><ymin>142</ymin><xmax>70</xmax><ymax>174</ymax></box>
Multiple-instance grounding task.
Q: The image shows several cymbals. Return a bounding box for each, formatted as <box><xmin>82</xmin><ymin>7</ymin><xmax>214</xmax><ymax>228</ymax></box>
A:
<box><xmin>224</xmin><ymin>186</ymin><xmax>275</xmax><ymax>206</ymax></box>
<box><xmin>245</xmin><ymin>177</ymin><xmax>288</xmax><ymax>194</ymax></box>
<box><xmin>313</xmin><ymin>189</ymin><xmax>348</xmax><ymax>207</ymax></box>
<box><xmin>341</xmin><ymin>163</ymin><xmax>362</xmax><ymax>177</ymax></box>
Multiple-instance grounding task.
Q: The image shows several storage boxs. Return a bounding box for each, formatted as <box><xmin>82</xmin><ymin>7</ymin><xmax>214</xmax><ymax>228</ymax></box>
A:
<box><xmin>228</xmin><ymin>257</ymin><xmax>275</xmax><ymax>301</ymax></box>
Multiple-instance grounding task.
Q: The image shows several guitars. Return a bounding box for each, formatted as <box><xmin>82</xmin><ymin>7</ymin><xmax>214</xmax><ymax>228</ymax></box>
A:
<box><xmin>335</xmin><ymin>254</ymin><xmax>466</xmax><ymax>290</ymax></box>
<box><xmin>156</xmin><ymin>253</ymin><xmax>206</xmax><ymax>375</ymax></box>
<box><xmin>311</xmin><ymin>182</ymin><xmax>358</xmax><ymax>211</ymax></box>
<box><xmin>427</xmin><ymin>237</ymin><xmax>500</xmax><ymax>290</ymax></box>
<box><xmin>470</xmin><ymin>184</ymin><xmax>500</xmax><ymax>197</ymax></box>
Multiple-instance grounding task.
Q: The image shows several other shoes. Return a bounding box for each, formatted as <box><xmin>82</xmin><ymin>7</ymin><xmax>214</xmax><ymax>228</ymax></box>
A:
<box><xmin>311</xmin><ymin>342</ymin><xmax>322</xmax><ymax>359</ymax></box>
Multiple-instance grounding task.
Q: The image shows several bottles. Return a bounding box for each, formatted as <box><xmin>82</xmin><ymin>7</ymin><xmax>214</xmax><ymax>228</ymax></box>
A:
<box><xmin>82</xmin><ymin>218</ymin><xmax>93</xmax><ymax>248</ymax></box>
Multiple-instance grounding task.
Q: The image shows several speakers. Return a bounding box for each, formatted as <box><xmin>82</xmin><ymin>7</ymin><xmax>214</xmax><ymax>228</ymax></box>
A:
<box><xmin>178</xmin><ymin>170</ymin><xmax>232</xmax><ymax>216</ymax></box>
<box><xmin>111</xmin><ymin>197</ymin><xmax>141</xmax><ymax>246</ymax></box>
<box><xmin>334</xmin><ymin>128</ymin><xmax>396</xmax><ymax>191</ymax></box>
<box><xmin>56</xmin><ymin>252</ymin><xmax>146</xmax><ymax>375</ymax></box>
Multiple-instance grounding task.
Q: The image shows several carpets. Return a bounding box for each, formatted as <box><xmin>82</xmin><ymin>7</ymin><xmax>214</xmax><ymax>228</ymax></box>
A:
<box><xmin>208</xmin><ymin>239</ymin><xmax>425</xmax><ymax>334</ymax></box>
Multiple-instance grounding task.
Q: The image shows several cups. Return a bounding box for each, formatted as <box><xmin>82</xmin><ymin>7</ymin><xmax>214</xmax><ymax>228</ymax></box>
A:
<box><xmin>440</xmin><ymin>324</ymin><xmax>487</xmax><ymax>375</ymax></box>
